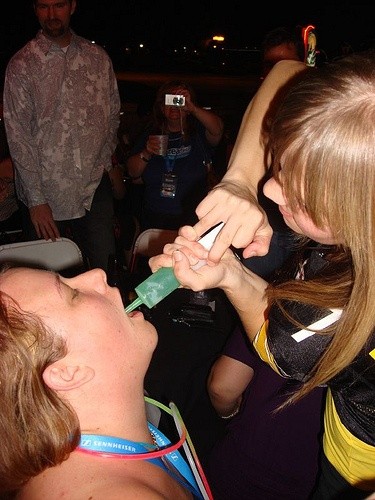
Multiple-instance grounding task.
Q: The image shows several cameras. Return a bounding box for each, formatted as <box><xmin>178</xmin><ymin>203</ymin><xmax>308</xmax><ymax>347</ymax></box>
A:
<box><xmin>165</xmin><ymin>94</ymin><xmax>186</xmax><ymax>106</ymax></box>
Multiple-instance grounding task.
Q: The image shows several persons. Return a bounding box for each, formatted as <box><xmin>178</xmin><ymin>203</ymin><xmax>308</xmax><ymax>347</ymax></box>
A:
<box><xmin>0</xmin><ymin>0</ymin><xmax>375</xmax><ymax>500</ymax></box>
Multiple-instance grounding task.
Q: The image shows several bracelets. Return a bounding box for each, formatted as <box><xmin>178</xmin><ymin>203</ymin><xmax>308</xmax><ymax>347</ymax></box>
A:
<box><xmin>219</xmin><ymin>408</ymin><xmax>239</xmax><ymax>419</ymax></box>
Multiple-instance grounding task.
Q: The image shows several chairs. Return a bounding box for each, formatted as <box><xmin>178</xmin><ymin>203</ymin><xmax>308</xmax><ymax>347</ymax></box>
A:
<box><xmin>0</xmin><ymin>216</ymin><xmax>180</xmax><ymax>278</ymax></box>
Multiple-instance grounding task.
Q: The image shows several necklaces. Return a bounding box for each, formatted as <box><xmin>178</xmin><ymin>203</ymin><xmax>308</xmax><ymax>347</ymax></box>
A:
<box><xmin>149</xmin><ymin>431</ymin><xmax>157</xmax><ymax>446</ymax></box>
<box><xmin>74</xmin><ymin>395</ymin><xmax>213</xmax><ymax>500</ymax></box>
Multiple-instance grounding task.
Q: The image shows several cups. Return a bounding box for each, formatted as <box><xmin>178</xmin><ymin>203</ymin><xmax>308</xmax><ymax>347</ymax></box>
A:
<box><xmin>148</xmin><ymin>135</ymin><xmax>168</xmax><ymax>157</ymax></box>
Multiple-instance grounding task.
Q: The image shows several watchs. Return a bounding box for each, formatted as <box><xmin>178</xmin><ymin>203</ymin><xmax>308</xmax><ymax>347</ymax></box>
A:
<box><xmin>140</xmin><ymin>151</ymin><xmax>150</xmax><ymax>163</ymax></box>
